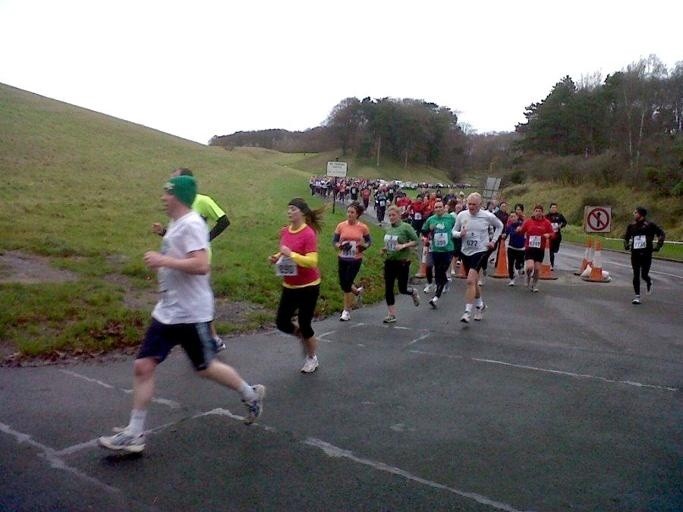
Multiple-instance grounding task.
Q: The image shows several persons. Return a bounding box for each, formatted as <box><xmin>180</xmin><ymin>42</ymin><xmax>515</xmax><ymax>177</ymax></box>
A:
<box><xmin>93</xmin><ymin>173</ymin><xmax>267</xmax><ymax>459</ymax></box>
<box><xmin>419</xmin><ymin>198</ymin><xmax>457</xmax><ymax>310</ymax></box>
<box><xmin>307</xmin><ymin>171</ymin><xmax>568</xmax><ymax>293</ymax></box>
<box><xmin>380</xmin><ymin>205</ymin><xmax>422</xmax><ymax>326</ymax></box>
<box><xmin>264</xmin><ymin>197</ymin><xmax>331</xmax><ymax>375</ymax></box>
<box><xmin>330</xmin><ymin>201</ymin><xmax>374</xmax><ymax>320</ymax></box>
<box><xmin>148</xmin><ymin>166</ymin><xmax>232</xmax><ymax>355</ymax></box>
<box><xmin>450</xmin><ymin>191</ymin><xmax>505</xmax><ymax>325</ymax></box>
<box><xmin>622</xmin><ymin>207</ymin><xmax>666</xmax><ymax>305</ymax></box>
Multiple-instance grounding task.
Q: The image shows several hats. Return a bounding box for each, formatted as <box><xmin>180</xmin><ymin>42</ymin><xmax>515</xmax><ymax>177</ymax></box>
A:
<box><xmin>162</xmin><ymin>174</ymin><xmax>199</xmax><ymax>210</ymax></box>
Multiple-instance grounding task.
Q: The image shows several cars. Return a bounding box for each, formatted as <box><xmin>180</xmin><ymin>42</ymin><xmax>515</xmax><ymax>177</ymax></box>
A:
<box><xmin>373</xmin><ymin>179</ymin><xmax>475</xmax><ymax>192</ymax></box>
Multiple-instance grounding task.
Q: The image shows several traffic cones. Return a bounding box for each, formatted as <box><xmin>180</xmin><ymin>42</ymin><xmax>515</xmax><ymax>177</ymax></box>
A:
<box><xmin>452</xmin><ymin>248</ymin><xmax>466</xmax><ymax>279</ymax></box>
<box><xmin>412</xmin><ymin>236</ymin><xmax>438</xmax><ymax>279</ymax></box>
<box><xmin>533</xmin><ymin>236</ymin><xmax>559</xmax><ymax>281</ymax></box>
<box><xmin>572</xmin><ymin>237</ymin><xmax>616</xmax><ymax>283</ymax></box>
<box><xmin>489</xmin><ymin>236</ymin><xmax>512</xmax><ymax>280</ymax></box>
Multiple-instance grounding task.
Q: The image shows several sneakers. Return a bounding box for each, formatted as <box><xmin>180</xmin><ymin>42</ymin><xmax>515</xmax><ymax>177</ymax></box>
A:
<box><xmin>382</xmin><ymin>314</ymin><xmax>397</xmax><ymax>324</ymax></box>
<box><xmin>423</xmin><ymin>257</ymin><xmax>555</xmax><ymax>294</ymax></box>
<box><xmin>473</xmin><ymin>302</ymin><xmax>488</xmax><ymax>321</ymax></box>
<box><xmin>338</xmin><ymin>310</ymin><xmax>350</xmax><ymax>323</ymax></box>
<box><xmin>428</xmin><ymin>297</ymin><xmax>438</xmax><ymax>310</ymax></box>
<box><xmin>460</xmin><ymin>308</ymin><xmax>472</xmax><ymax>324</ymax></box>
<box><xmin>631</xmin><ymin>295</ymin><xmax>643</xmax><ymax>306</ymax></box>
<box><xmin>299</xmin><ymin>354</ymin><xmax>319</xmax><ymax>375</ymax></box>
<box><xmin>96</xmin><ymin>429</ymin><xmax>147</xmax><ymax>454</ymax></box>
<box><xmin>355</xmin><ymin>286</ymin><xmax>364</xmax><ymax>305</ymax></box>
<box><xmin>208</xmin><ymin>335</ymin><xmax>228</xmax><ymax>354</ymax></box>
<box><xmin>410</xmin><ymin>289</ymin><xmax>420</xmax><ymax>307</ymax></box>
<box><xmin>646</xmin><ymin>279</ymin><xmax>655</xmax><ymax>297</ymax></box>
<box><xmin>239</xmin><ymin>384</ymin><xmax>267</xmax><ymax>426</ymax></box>
